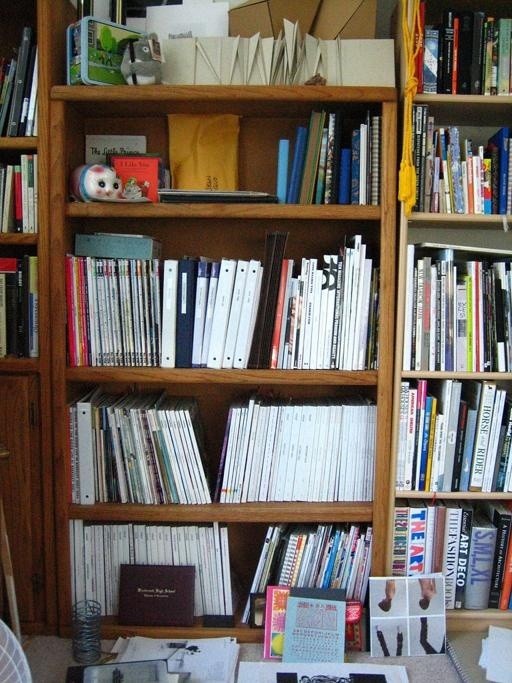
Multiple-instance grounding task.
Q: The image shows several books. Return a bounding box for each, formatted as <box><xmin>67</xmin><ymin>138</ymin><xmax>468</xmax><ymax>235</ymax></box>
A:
<box><xmin>264</xmin><ymin>585</ymin><xmax>346</xmax><ymax>662</ymax></box>
<box><xmin>277</xmin><ymin>110</ymin><xmax>379</xmax><ymax>206</ymax></box>
<box><xmin>413</xmin><ymin>1</ymin><xmax>511</xmax><ymax>96</ymax></box>
<box><xmin>369</xmin><ymin>572</ymin><xmax>446</xmax><ymax>657</ymax></box>
<box><xmin>393</xmin><ymin>499</ymin><xmax>511</xmax><ymax>610</ymax></box>
<box><xmin>402</xmin><ymin>242</ymin><xmax>511</xmax><ymax>372</ymax></box>
<box><xmin>395</xmin><ymin>377</ymin><xmax>511</xmax><ymax>493</ymax></box>
<box><xmin>412</xmin><ymin>103</ymin><xmax>511</xmax><ymax>215</ymax></box>
<box><xmin>69</xmin><ymin>518</ymin><xmax>244</xmax><ymax>621</ymax></box>
<box><xmin>66</xmin><ymin>230</ymin><xmax>379</xmax><ymax>370</ymax></box>
<box><xmin>242</xmin><ymin>525</ymin><xmax>373</xmax><ymax>627</ymax></box>
<box><xmin>69</xmin><ymin>390</ymin><xmax>377</xmax><ymax>504</ymax></box>
<box><xmin>1</xmin><ymin>28</ymin><xmax>39</xmax><ymax>360</ymax></box>
<box><xmin>444</xmin><ymin>631</ymin><xmax>497</xmax><ymax>683</ymax></box>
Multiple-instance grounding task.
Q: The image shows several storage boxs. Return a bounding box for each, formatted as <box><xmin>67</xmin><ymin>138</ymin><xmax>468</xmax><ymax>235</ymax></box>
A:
<box><xmin>65</xmin><ymin>15</ymin><xmax>147</xmax><ymax>86</ymax></box>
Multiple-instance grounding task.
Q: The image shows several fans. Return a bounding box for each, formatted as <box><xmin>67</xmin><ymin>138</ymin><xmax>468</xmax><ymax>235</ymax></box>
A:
<box><xmin>0</xmin><ymin>617</ymin><xmax>33</xmax><ymax>683</ymax></box>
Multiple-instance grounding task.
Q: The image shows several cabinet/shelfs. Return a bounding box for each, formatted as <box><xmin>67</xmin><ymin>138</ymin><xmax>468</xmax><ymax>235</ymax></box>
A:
<box><xmin>0</xmin><ymin>0</ymin><xmax>59</xmax><ymax>638</ymax></box>
<box><xmin>385</xmin><ymin>0</ymin><xmax>512</xmax><ymax>629</ymax></box>
<box><xmin>47</xmin><ymin>86</ymin><xmax>398</xmax><ymax>640</ymax></box>
